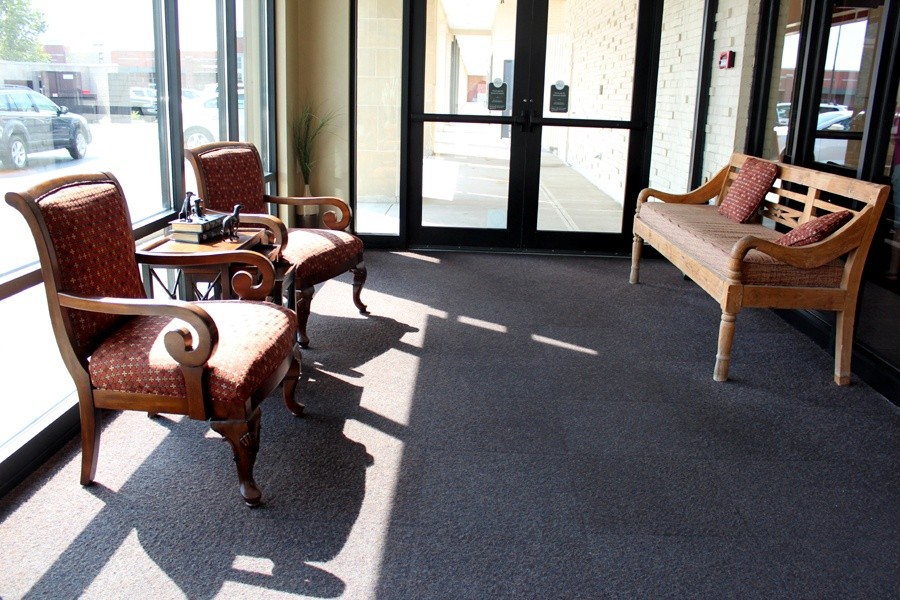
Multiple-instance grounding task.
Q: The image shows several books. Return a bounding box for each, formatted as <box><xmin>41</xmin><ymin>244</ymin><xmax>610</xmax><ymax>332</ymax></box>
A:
<box><xmin>167</xmin><ymin>213</ymin><xmax>233</xmax><ymax>244</ymax></box>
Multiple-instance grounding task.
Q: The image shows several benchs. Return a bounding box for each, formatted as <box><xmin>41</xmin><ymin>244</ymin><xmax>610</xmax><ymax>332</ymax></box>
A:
<box><xmin>630</xmin><ymin>153</ymin><xmax>890</xmax><ymax>388</ymax></box>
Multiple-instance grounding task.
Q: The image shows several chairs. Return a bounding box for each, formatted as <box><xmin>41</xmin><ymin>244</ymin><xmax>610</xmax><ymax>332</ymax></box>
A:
<box><xmin>3</xmin><ymin>172</ymin><xmax>305</xmax><ymax>508</ymax></box>
<box><xmin>182</xmin><ymin>140</ymin><xmax>367</xmax><ymax>347</ymax></box>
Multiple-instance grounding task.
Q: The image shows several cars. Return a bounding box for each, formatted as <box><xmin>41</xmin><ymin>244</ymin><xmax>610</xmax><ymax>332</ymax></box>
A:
<box><xmin>129</xmin><ymin>86</ymin><xmax>157</xmax><ymax>115</ymax></box>
<box><xmin>771</xmin><ymin>101</ymin><xmax>853</xmax><ymax>169</ymax></box>
<box><xmin>0</xmin><ymin>84</ymin><xmax>93</xmax><ymax>171</ymax></box>
<box><xmin>171</xmin><ymin>92</ymin><xmax>245</xmax><ymax>154</ymax></box>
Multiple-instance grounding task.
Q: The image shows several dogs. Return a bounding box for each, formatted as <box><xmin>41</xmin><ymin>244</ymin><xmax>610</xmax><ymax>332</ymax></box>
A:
<box><xmin>179</xmin><ymin>191</ymin><xmax>196</xmax><ymax>221</ymax></box>
<box><xmin>220</xmin><ymin>205</ymin><xmax>242</xmax><ymax>242</ymax></box>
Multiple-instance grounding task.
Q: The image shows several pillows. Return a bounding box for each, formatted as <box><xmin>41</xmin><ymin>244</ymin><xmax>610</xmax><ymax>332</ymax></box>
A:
<box><xmin>720</xmin><ymin>157</ymin><xmax>780</xmax><ymax>225</ymax></box>
<box><xmin>775</xmin><ymin>208</ymin><xmax>854</xmax><ymax>246</ymax></box>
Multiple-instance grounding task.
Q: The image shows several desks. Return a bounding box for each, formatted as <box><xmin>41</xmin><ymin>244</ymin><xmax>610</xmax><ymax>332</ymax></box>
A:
<box><xmin>140</xmin><ymin>228</ymin><xmax>261</xmax><ymax>305</ymax></box>
